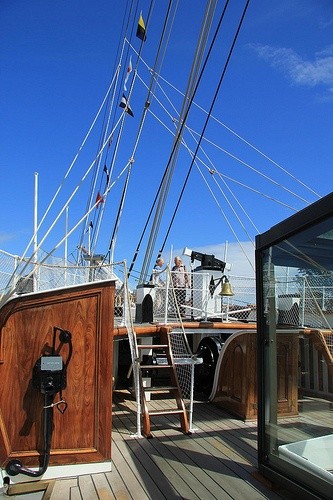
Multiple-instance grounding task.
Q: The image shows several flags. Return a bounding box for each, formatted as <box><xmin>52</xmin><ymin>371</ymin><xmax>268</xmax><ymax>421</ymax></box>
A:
<box><xmin>103</xmin><ymin>164</ymin><xmax>110</xmax><ymax>185</ymax></box>
<box><xmin>123</xmin><ymin>54</ymin><xmax>132</xmax><ymax>91</ymax></box>
<box><xmin>95</xmin><ymin>192</ymin><xmax>104</xmax><ymax>209</ymax></box>
<box><xmin>118</xmin><ymin>93</ymin><xmax>134</xmax><ymax>118</ymax></box>
<box><xmin>136</xmin><ymin>13</ymin><xmax>147</xmax><ymax>42</ymax></box>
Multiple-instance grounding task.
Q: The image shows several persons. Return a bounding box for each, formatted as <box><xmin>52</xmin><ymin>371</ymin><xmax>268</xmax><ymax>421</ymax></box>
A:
<box><xmin>170</xmin><ymin>256</ymin><xmax>191</xmax><ymax>321</ymax></box>
<box><xmin>152</xmin><ymin>258</ymin><xmax>169</xmax><ymax>314</ymax></box>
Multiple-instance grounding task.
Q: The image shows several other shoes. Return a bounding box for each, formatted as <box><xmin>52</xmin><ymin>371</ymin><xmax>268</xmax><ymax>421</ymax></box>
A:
<box><xmin>181</xmin><ymin>315</ymin><xmax>186</xmax><ymax>317</ymax></box>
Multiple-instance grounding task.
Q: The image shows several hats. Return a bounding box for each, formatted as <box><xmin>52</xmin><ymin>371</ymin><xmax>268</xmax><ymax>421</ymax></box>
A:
<box><xmin>158</xmin><ymin>258</ymin><xmax>164</xmax><ymax>262</ymax></box>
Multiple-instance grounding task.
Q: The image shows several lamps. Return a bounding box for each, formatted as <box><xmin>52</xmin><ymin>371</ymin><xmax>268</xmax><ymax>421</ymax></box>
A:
<box><xmin>210</xmin><ymin>274</ymin><xmax>234</xmax><ymax>297</ymax></box>
